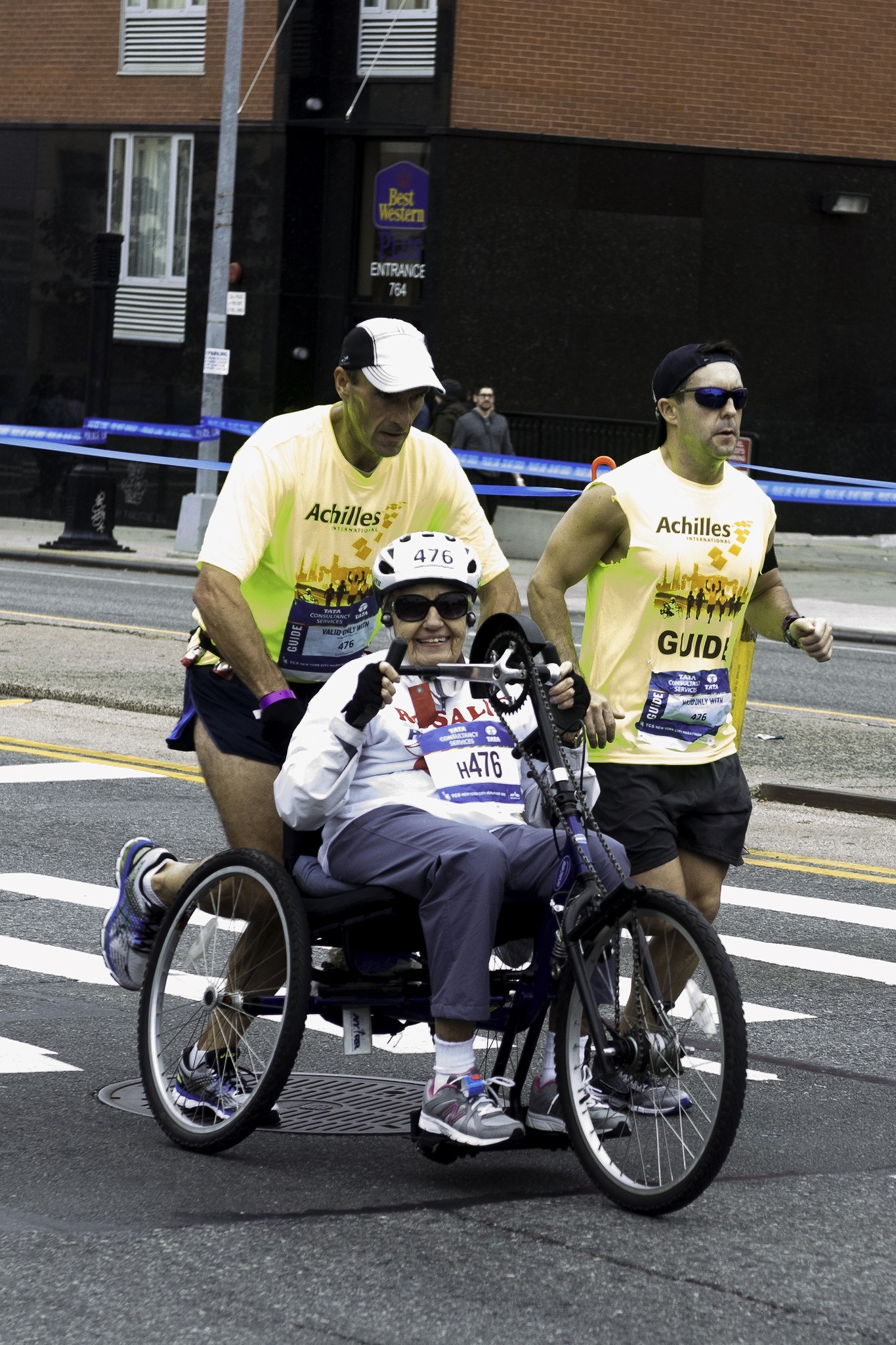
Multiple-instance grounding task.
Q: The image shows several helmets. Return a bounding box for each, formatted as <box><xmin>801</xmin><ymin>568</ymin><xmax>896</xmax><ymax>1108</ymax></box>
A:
<box><xmin>371</xmin><ymin>531</ymin><xmax>484</xmax><ymax>608</ymax></box>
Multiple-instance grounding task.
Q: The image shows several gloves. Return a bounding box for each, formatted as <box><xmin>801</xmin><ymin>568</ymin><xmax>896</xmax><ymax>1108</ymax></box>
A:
<box><xmin>258</xmin><ymin>691</ymin><xmax>305</xmax><ymax>757</ymax></box>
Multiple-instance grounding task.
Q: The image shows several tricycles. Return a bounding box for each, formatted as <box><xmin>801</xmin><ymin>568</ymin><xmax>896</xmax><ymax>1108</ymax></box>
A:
<box><xmin>136</xmin><ymin>606</ymin><xmax>751</xmax><ymax>1219</ymax></box>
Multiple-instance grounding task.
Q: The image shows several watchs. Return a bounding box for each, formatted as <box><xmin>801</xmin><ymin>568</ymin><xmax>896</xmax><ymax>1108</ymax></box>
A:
<box><xmin>781</xmin><ymin>613</ymin><xmax>806</xmax><ymax>650</ymax></box>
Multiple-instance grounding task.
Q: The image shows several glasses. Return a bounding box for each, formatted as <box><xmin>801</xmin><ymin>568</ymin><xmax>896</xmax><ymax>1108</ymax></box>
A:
<box><xmin>392</xmin><ymin>590</ymin><xmax>471</xmax><ymax>623</ymax></box>
<box><xmin>654</xmin><ymin>386</ymin><xmax>749</xmax><ymax>410</ymax></box>
<box><xmin>479</xmin><ymin>394</ymin><xmax>495</xmax><ymax>398</ymax></box>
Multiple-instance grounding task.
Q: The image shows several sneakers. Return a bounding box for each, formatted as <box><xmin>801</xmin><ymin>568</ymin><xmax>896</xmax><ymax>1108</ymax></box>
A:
<box><xmin>525</xmin><ymin>1065</ymin><xmax>632</xmax><ymax>1139</ymax></box>
<box><xmin>590</xmin><ymin>1058</ymin><xmax>692</xmax><ymax>1115</ymax></box>
<box><xmin>171</xmin><ymin>1046</ymin><xmax>281</xmax><ymax>1126</ymax></box>
<box><xmin>417</xmin><ymin>1067</ymin><xmax>525</xmax><ymax>1148</ymax></box>
<box><xmin>99</xmin><ymin>837</ymin><xmax>178</xmax><ymax>992</ymax></box>
<box><xmin>495</xmin><ymin>938</ymin><xmax>535</xmax><ymax>968</ymax></box>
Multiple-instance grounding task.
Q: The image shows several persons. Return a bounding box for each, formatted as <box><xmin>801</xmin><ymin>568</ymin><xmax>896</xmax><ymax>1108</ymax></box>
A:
<box><xmin>527</xmin><ymin>340</ymin><xmax>834</xmax><ymax>1119</ymax></box>
<box><xmin>271</xmin><ymin>533</ymin><xmax>633</xmax><ymax>1149</ymax></box>
<box><xmin>100</xmin><ymin>318</ymin><xmax>522</xmax><ymax>1125</ymax></box>
<box><xmin>412</xmin><ymin>379</ymin><xmax>524</xmax><ymax>527</ymax></box>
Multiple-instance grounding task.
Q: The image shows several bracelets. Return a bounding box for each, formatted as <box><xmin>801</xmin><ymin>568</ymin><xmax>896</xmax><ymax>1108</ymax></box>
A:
<box><xmin>253</xmin><ymin>688</ymin><xmax>296</xmax><ymax>720</ymax></box>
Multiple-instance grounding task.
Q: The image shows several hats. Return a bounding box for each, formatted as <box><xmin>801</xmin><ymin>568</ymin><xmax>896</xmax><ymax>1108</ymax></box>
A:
<box><xmin>651</xmin><ymin>344</ymin><xmax>740</xmax><ymax>449</ymax></box>
<box><xmin>337</xmin><ymin>317</ymin><xmax>446</xmax><ymax>396</ymax></box>
